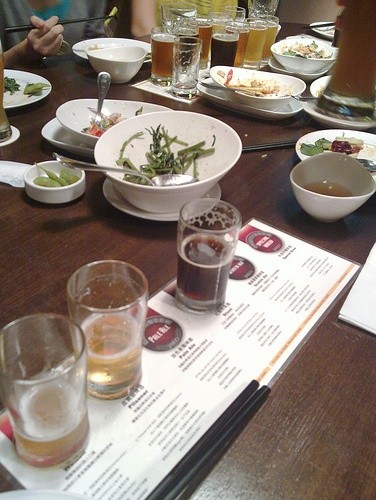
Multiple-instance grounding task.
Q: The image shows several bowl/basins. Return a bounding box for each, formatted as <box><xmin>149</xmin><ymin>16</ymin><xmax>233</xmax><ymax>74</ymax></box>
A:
<box><xmin>94</xmin><ymin>112</ymin><xmax>242</xmax><ymax>215</ymax></box>
<box><xmin>209</xmin><ymin>63</ymin><xmax>307</xmax><ymax>108</ymax></box>
<box><xmin>270</xmin><ymin>36</ymin><xmax>336</xmax><ymax>74</ymax></box>
<box><xmin>289</xmin><ymin>153</ymin><xmax>376</xmax><ymax>223</ymax></box>
<box><xmin>86</xmin><ymin>44</ymin><xmax>146</xmax><ymax>84</ymax></box>
<box><xmin>24</xmin><ymin>162</ymin><xmax>87</xmax><ymax>203</ymax></box>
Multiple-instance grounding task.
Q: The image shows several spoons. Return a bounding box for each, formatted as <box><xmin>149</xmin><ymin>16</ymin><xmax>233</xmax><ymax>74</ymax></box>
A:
<box><xmin>51</xmin><ymin>151</ymin><xmax>199</xmax><ymax>187</ymax></box>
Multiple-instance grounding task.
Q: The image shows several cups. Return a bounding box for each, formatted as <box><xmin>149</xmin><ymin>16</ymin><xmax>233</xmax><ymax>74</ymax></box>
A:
<box><xmin>315</xmin><ymin>1</ymin><xmax>376</xmax><ymax>124</ymax></box>
<box><xmin>0</xmin><ymin>39</ymin><xmax>14</xmax><ymax>143</ymax></box>
<box><xmin>149</xmin><ymin>0</ymin><xmax>282</xmax><ymax>100</ymax></box>
<box><xmin>0</xmin><ymin>314</ymin><xmax>88</xmax><ymax>468</ymax></box>
<box><xmin>66</xmin><ymin>258</ymin><xmax>150</xmax><ymax>400</ymax></box>
<box><xmin>175</xmin><ymin>198</ymin><xmax>242</xmax><ymax>312</ymax></box>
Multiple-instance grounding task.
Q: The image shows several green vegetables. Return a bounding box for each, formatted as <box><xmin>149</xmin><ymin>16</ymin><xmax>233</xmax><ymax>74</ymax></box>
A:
<box><xmin>300</xmin><ymin>138</ymin><xmax>333</xmax><ymax>156</ymax></box>
<box><xmin>3</xmin><ymin>76</ymin><xmax>49</xmax><ymax>96</ymax></box>
<box><xmin>117</xmin><ymin>126</ymin><xmax>216</xmax><ymax>186</ymax></box>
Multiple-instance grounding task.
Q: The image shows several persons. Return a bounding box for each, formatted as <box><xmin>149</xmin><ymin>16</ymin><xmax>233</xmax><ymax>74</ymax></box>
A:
<box><xmin>0</xmin><ymin>0</ymin><xmax>250</xmax><ymax>70</ymax></box>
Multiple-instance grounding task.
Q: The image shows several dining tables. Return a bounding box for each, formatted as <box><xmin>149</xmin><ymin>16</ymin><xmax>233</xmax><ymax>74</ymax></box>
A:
<box><xmin>0</xmin><ymin>23</ymin><xmax>376</xmax><ymax>500</ymax></box>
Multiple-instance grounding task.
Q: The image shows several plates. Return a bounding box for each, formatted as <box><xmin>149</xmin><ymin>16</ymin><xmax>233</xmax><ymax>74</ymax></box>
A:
<box><xmin>0</xmin><ymin>36</ymin><xmax>172</xmax><ymax>153</ymax></box>
<box><xmin>103</xmin><ymin>176</ymin><xmax>221</xmax><ymax>222</ymax></box>
<box><xmin>310</xmin><ymin>22</ymin><xmax>336</xmax><ymax>41</ymax></box>
<box><xmin>295</xmin><ymin>129</ymin><xmax>376</xmax><ymax>192</ymax></box>
<box><xmin>0</xmin><ymin>488</ymin><xmax>93</xmax><ymax>500</ymax></box>
<box><xmin>298</xmin><ymin>72</ymin><xmax>376</xmax><ymax>132</ymax></box>
<box><xmin>195</xmin><ymin>78</ymin><xmax>304</xmax><ymax>122</ymax></box>
<box><xmin>267</xmin><ymin>58</ymin><xmax>335</xmax><ymax>83</ymax></box>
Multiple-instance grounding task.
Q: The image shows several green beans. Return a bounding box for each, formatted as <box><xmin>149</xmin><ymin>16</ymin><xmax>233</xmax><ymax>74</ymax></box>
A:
<box><xmin>33</xmin><ymin>163</ymin><xmax>81</xmax><ymax>187</ymax></box>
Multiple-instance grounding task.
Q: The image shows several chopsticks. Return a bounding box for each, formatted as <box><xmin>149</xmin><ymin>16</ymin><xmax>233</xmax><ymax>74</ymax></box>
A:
<box><xmin>5</xmin><ymin>15</ymin><xmax>116</xmax><ymax>33</ymax></box>
<box><xmin>302</xmin><ymin>22</ymin><xmax>337</xmax><ymax>30</ymax></box>
<box><xmin>142</xmin><ymin>379</ymin><xmax>271</xmax><ymax>500</ymax></box>
<box><xmin>239</xmin><ymin>137</ymin><xmax>300</xmax><ymax>154</ymax></box>
